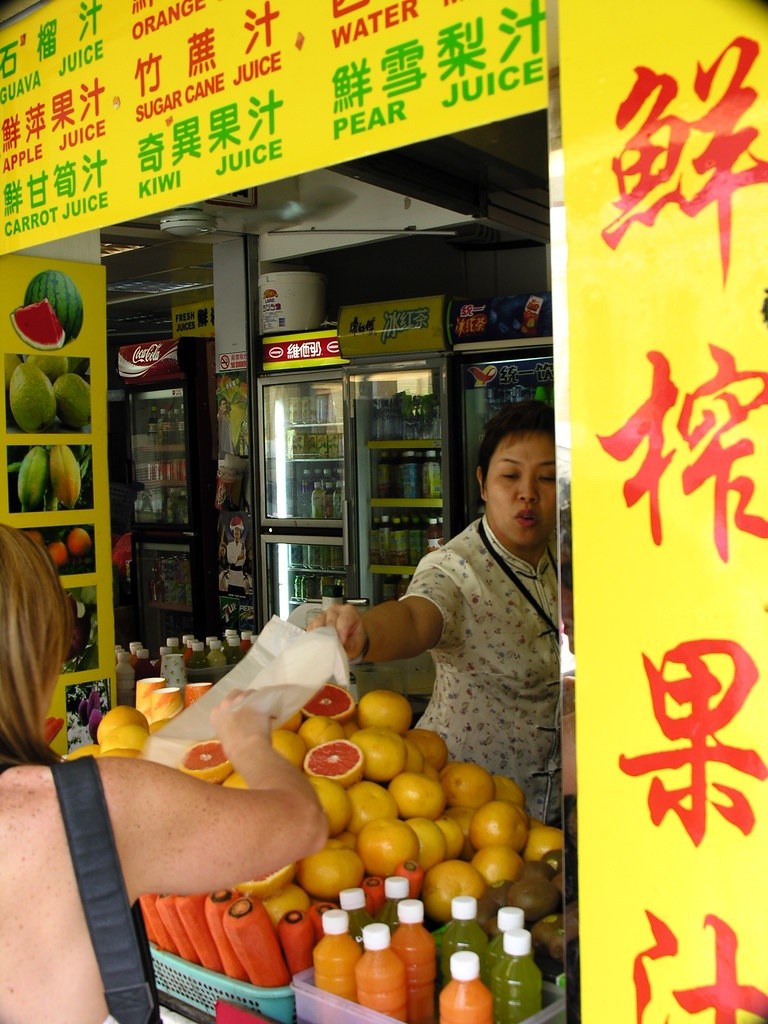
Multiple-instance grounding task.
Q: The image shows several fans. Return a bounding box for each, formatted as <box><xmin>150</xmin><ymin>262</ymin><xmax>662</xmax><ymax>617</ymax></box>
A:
<box><xmin>101</xmin><ymin>200</ymin><xmax>460</xmax><ymax>262</ymax></box>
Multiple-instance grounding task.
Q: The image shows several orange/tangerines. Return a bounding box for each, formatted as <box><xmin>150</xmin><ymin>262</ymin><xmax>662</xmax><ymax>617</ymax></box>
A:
<box><xmin>25</xmin><ymin>528</ymin><xmax>92</xmax><ymax>566</ymax></box>
<box><xmin>64</xmin><ymin>684</ymin><xmax>563</xmax><ymax>923</ymax></box>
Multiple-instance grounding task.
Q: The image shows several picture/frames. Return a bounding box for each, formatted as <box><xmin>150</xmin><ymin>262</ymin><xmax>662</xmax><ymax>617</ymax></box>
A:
<box><xmin>204</xmin><ymin>186</ymin><xmax>260</xmax><ymax>209</ymax></box>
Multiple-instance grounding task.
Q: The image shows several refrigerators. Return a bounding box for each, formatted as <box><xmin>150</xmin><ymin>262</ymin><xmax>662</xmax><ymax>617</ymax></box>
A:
<box><xmin>117</xmin><ymin>337</ymin><xmax>218</xmax><ymax>649</ymax></box>
<box><xmin>256</xmin><ymin>328</ymin><xmax>360</xmax><ymax>619</ymax></box>
<box><xmin>336</xmin><ymin>293</ymin><xmax>457</xmax><ymax>613</ymax></box>
<box><xmin>444</xmin><ymin>290</ymin><xmax>554</xmax><ymax>546</ymax></box>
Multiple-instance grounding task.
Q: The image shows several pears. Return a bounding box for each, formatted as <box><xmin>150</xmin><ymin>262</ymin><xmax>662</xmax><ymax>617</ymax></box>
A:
<box><xmin>3</xmin><ymin>354</ymin><xmax>91</xmax><ymax>433</ymax></box>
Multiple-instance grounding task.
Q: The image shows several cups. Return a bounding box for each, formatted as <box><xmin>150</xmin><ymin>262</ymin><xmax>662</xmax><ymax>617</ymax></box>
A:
<box><xmin>134</xmin><ymin>655</ymin><xmax>214</xmax><ymax>725</ymax></box>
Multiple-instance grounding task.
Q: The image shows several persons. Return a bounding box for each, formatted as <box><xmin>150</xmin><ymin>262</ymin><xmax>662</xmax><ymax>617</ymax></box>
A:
<box><xmin>307</xmin><ymin>399</ymin><xmax>562</xmax><ymax>825</ymax></box>
<box><xmin>0</xmin><ymin>524</ymin><xmax>330</xmax><ymax>1024</ymax></box>
<box><xmin>217</xmin><ymin>397</ymin><xmax>234</xmax><ymax>454</ymax></box>
<box><xmin>219</xmin><ymin>527</ymin><xmax>246</xmax><ymax>594</ymax></box>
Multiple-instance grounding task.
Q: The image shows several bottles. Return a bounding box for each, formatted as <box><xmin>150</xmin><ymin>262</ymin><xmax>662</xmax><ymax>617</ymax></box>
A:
<box><xmin>112</xmin><ymin>629</ymin><xmax>261</xmax><ymax>707</ymax></box>
<box><xmin>369</xmin><ymin>512</ymin><xmax>443</xmax><ymax>566</ymax></box>
<box><xmin>312</xmin><ymin>876</ymin><xmax>543</xmax><ymax>1024</ymax></box>
<box><xmin>486</xmin><ymin>384</ymin><xmax>554</xmax><ymax>420</ymax></box>
<box><xmin>153</xmin><ymin>555</ymin><xmax>192</xmax><ymax>607</ymax></box>
<box><xmin>377</xmin><ymin>450</ymin><xmax>442</xmax><ymax>500</ymax></box>
<box><xmin>398</xmin><ymin>575</ymin><xmax>410</xmax><ymax>598</ymax></box>
<box><xmin>296</xmin><ymin>468</ymin><xmax>342</xmax><ymax>519</ymax></box>
<box><xmin>165</xmin><ymin>491</ymin><xmax>188</xmax><ymax>524</ymax></box>
<box><xmin>147</xmin><ymin>402</ymin><xmax>184</xmax><ymax>445</ymax></box>
<box><xmin>382</xmin><ymin>574</ymin><xmax>395</xmax><ymax>601</ymax></box>
<box><xmin>153</xmin><ymin>458</ymin><xmax>185</xmax><ymax>480</ymax></box>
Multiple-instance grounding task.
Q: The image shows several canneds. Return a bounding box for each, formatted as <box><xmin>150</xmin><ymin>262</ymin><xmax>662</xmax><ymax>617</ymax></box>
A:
<box><xmin>146</xmin><ymin>457</ymin><xmax>185</xmax><ymax>481</ymax></box>
<box><xmin>285</xmin><ymin>394</ymin><xmax>350</xmax><ymax>600</ymax></box>
<box><xmin>150</xmin><ymin>555</ymin><xmax>192</xmax><ymax>606</ymax></box>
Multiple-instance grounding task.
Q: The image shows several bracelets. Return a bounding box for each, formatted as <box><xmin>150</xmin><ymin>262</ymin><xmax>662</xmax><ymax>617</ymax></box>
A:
<box><xmin>350</xmin><ymin>633</ymin><xmax>370</xmax><ymax>664</ymax></box>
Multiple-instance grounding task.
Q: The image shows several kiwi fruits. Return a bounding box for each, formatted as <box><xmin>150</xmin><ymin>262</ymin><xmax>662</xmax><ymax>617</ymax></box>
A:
<box><xmin>466</xmin><ymin>848</ymin><xmax>565</xmax><ymax>966</ymax></box>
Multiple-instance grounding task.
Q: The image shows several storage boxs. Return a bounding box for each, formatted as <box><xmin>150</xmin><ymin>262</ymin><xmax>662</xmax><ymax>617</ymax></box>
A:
<box><xmin>287</xmin><ymin>947</ymin><xmax>567</xmax><ymax>1024</ymax></box>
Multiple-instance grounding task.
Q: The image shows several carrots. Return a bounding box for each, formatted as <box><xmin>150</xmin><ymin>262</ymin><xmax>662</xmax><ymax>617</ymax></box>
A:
<box><xmin>129</xmin><ymin>859</ymin><xmax>425</xmax><ymax>989</ymax></box>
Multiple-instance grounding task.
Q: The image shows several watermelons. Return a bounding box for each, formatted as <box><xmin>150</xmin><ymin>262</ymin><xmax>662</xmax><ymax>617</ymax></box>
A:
<box><xmin>10</xmin><ymin>270</ymin><xmax>83</xmax><ymax>351</ymax></box>
